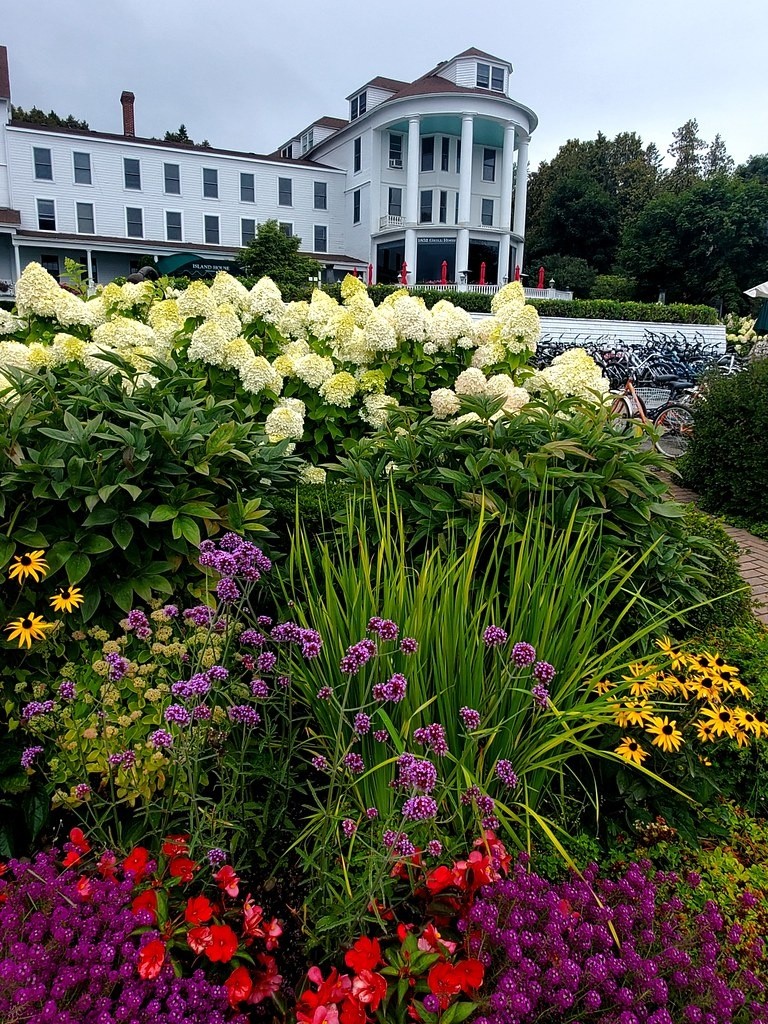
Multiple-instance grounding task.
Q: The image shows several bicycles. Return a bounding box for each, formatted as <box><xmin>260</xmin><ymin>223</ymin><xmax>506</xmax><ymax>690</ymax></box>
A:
<box><xmin>526</xmin><ymin>328</ymin><xmax>747</xmax><ymax>459</ymax></box>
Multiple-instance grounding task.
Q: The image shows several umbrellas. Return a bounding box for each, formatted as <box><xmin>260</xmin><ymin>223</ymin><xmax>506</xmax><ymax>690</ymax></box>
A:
<box><xmin>401</xmin><ymin>261</ymin><xmax>408</xmax><ymax>285</ymax></box>
<box><xmin>353</xmin><ymin>266</ymin><xmax>357</xmax><ymax>277</ymax></box>
<box><xmin>743</xmin><ymin>281</ymin><xmax>768</xmax><ymax>340</ymax></box>
<box><xmin>154</xmin><ymin>254</ymin><xmax>203</xmax><ymax>278</ymax></box>
<box><xmin>480</xmin><ymin>262</ymin><xmax>486</xmax><ymax>284</ymax></box>
<box><xmin>537</xmin><ymin>267</ymin><xmax>544</xmax><ymax>289</ymax></box>
<box><xmin>515</xmin><ymin>265</ymin><xmax>520</xmax><ymax>281</ymax></box>
<box><xmin>442</xmin><ymin>261</ymin><xmax>447</xmax><ymax>285</ymax></box>
<box><xmin>369</xmin><ymin>264</ymin><xmax>373</xmax><ymax>285</ymax></box>
